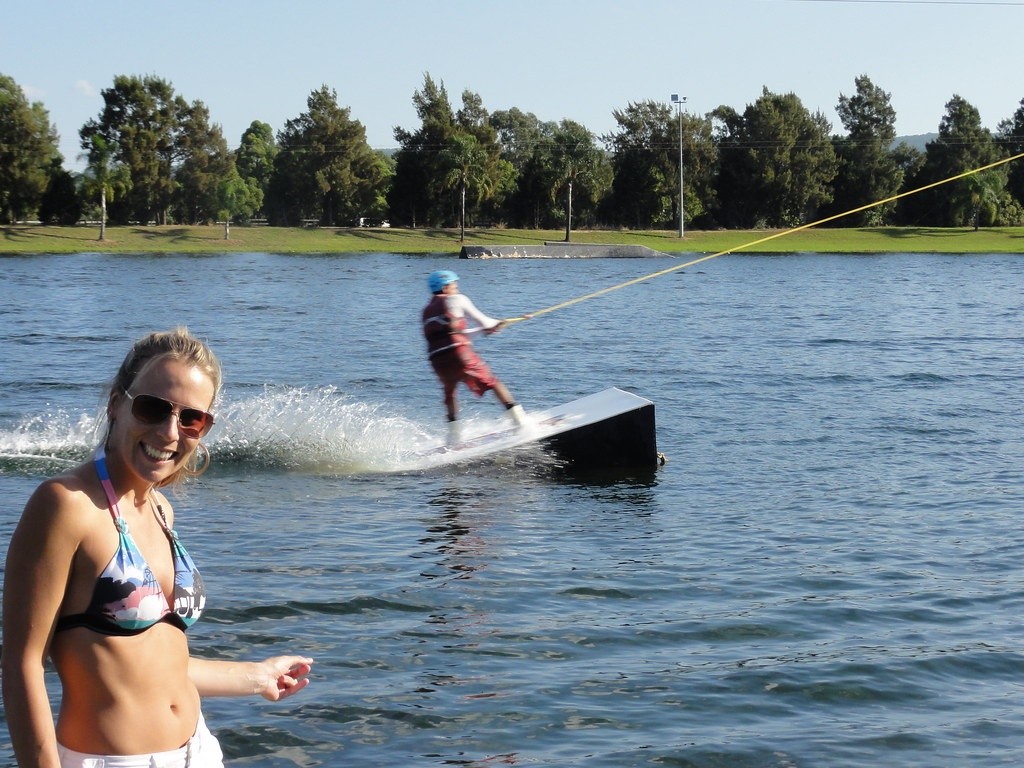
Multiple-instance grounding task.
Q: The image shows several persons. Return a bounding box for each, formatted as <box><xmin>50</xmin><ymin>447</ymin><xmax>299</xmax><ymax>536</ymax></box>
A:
<box><xmin>0</xmin><ymin>331</ymin><xmax>314</xmax><ymax>768</ymax></box>
<box><xmin>421</xmin><ymin>270</ymin><xmax>527</xmax><ymax>443</ymax></box>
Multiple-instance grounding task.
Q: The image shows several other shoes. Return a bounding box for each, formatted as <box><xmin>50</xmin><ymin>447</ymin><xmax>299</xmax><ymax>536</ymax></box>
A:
<box><xmin>507</xmin><ymin>404</ymin><xmax>528</xmax><ymax>426</ymax></box>
<box><xmin>446</xmin><ymin>420</ymin><xmax>465</xmax><ymax>442</ymax></box>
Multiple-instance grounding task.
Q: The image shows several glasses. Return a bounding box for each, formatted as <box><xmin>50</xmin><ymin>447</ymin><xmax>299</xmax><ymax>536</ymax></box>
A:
<box><xmin>120</xmin><ymin>384</ymin><xmax>215</xmax><ymax>439</ymax></box>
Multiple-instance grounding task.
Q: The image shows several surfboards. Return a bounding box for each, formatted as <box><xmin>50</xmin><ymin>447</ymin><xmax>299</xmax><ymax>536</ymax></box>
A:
<box><xmin>425</xmin><ymin>411</ymin><xmax>570</xmax><ymax>456</ymax></box>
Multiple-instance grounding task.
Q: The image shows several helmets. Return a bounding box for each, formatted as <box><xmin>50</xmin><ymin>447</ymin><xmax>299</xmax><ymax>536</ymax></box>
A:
<box><xmin>429</xmin><ymin>270</ymin><xmax>460</xmax><ymax>293</ymax></box>
